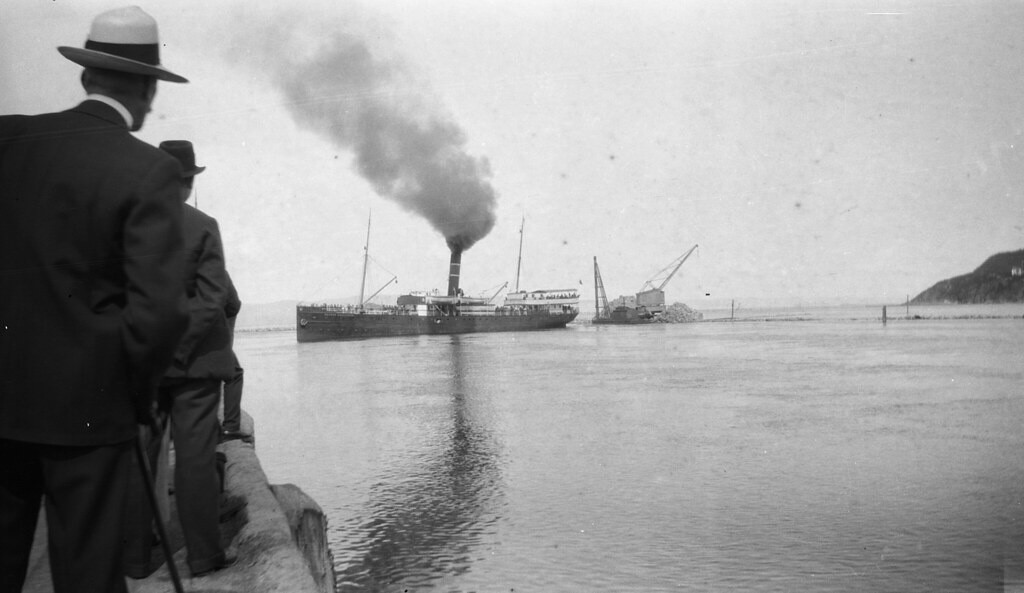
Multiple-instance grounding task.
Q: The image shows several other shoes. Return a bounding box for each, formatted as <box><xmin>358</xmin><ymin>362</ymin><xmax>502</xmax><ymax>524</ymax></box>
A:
<box><xmin>191</xmin><ymin>553</ymin><xmax>240</xmax><ymax>577</ymax></box>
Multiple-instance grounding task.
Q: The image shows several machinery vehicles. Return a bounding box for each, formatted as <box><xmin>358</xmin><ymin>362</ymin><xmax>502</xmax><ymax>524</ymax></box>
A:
<box><xmin>632</xmin><ymin>243</ymin><xmax>700</xmax><ymax>318</ymax></box>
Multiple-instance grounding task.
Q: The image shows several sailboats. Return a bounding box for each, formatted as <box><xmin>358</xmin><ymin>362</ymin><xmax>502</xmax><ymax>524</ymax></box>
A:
<box><xmin>296</xmin><ymin>207</ymin><xmax>583</xmax><ymax>344</ymax></box>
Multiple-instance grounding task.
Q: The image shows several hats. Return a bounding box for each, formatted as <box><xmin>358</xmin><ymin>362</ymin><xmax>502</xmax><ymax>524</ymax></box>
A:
<box><xmin>56</xmin><ymin>5</ymin><xmax>190</xmax><ymax>83</ymax></box>
<box><xmin>158</xmin><ymin>140</ymin><xmax>206</xmax><ymax>178</ymax></box>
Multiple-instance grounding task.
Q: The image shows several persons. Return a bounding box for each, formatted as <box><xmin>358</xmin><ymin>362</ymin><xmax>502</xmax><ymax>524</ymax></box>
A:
<box><xmin>0</xmin><ymin>3</ymin><xmax>251</xmax><ymax>593</ymax></box>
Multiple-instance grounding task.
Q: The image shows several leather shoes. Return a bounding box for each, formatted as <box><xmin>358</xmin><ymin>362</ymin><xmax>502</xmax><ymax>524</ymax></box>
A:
<box><xmin>220</xmin><ymin>429</ymin><xmax>251</xmax><ymax>441</ymax></box>
